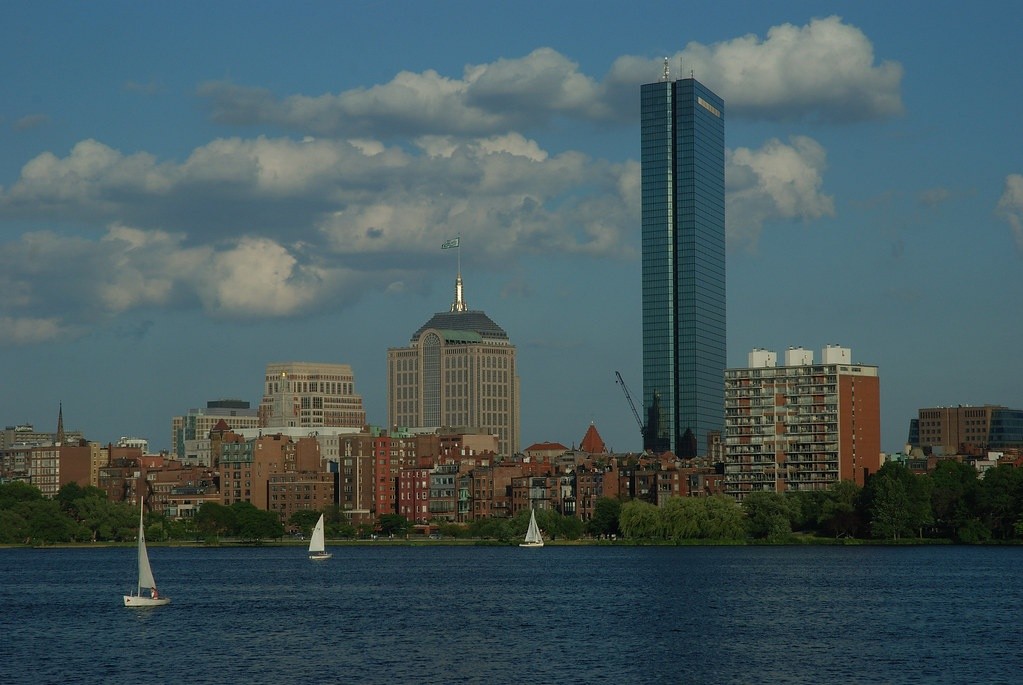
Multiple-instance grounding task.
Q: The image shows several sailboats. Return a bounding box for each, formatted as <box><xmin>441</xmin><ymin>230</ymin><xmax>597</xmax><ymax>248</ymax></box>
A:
<box><xmin>307</xmin><ymin>513</ymin><xmax>333</xmax><ymax>560</ymax></box>
<box><xmin>519</xmin><ymin>508</ymin><xmax>545</xmax><ymax>548</ymax></box>
<box><xmin>123</xmin><ymin>495</ymin><xmax>171</xmax><ymax>607</ymax></box>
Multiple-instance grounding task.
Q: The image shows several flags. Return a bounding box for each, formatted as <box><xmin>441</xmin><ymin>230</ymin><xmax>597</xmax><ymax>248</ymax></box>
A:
<box><xmin>441</xmin><ymin>237</ymin><xmax>459</xmax><ymax>249</ymax></box>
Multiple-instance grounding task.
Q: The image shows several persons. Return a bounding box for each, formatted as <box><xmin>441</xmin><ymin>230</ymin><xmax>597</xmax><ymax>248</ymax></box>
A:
<box><xmin>151</xmin><ymin>587</ymin><xmax>159</xmax><ymax>600</ymax></box>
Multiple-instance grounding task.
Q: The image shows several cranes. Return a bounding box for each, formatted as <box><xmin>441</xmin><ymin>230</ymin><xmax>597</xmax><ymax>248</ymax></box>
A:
<box><xmin>615</xmin><ymin>370</ymin><xmax>644</xmax><ymax>437</ymax></box>
